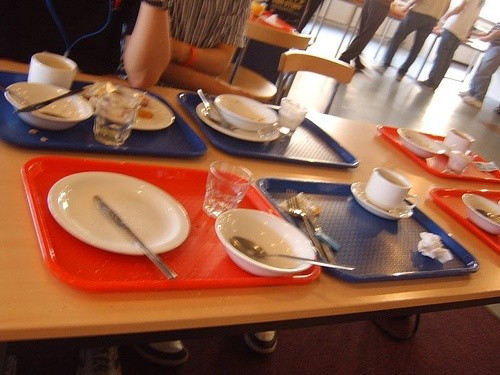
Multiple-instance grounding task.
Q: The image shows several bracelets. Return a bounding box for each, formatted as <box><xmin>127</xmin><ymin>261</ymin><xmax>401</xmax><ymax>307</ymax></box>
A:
<box><xmin>144</xmin><ymin>0</ymin><xmax>172</xmax><ymax>10</ymax></box>
<box><xmin>181</xmin><ymin>45</ymin><xmax>197</xmax><ymax>67</ymax></box>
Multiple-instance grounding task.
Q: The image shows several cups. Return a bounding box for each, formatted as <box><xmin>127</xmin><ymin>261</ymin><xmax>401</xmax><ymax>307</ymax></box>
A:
<box><xmin>278</xmin><ymin>97</ymin><xmax>309</xmax><ymax>135</ymax></box>
<box><xmin>444</xmin><ymin>128</ymin><xmax>475</xmax><ymax>155</ymax></box>
<box><xmin>92</xmin><ymin>93</ymin><xmax>138</xmax><ymax>147</ymax></box>
<box><xmin>27</xmin><ymin>54</ymin><xmax>77</xmax><ymax>91</ymax></box>
<box><xmin>203</xmin><ymin>161</ymin><xmax>253</xmax><ymax>222</ymax></box>
<box><xmin>449</xmin><ymin>144</ymin><xmax>474</xmax><ymax>172</ymax></box>
<box><xmin>365</xmin><ymin>167</ymin><xmax>419</xmax><ymax>213</ymax></box>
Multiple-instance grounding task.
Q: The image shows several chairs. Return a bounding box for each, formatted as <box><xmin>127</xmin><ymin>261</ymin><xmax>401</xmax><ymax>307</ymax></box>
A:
<box><xmin>217</xmin><ymin>25</ymin><xmax>353</xmax><ymax>115</ymax></box>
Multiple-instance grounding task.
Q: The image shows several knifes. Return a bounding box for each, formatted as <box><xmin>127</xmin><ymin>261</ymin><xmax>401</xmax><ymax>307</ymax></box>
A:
<box><xmin>94</xmin><ymin>195</ymin><xmax>176</xmax><ymax>280</ymax></box>
<box><xmin>13</xmin><ymin>89</ymin><xmax>86</xmax><ymax>113</ymax></box>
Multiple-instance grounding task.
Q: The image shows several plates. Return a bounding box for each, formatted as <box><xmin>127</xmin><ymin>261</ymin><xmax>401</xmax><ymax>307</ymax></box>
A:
<box><xmin>351</xmin><ymin>181</ymin><xmax>414</xmax><ymax>220</ymax></box>
<box><xmin>47</xmin><ymin>172</ymin><xmax>189</xmax><ymax>255</ymax></box>
<box><xmin>89</xmin><ymin>87</ymin><xmax>175</xmax><ymax>131</ymax></box>
<box><xmin>196</xmin><ymin>101</ymin><xmax>281</xmax><ymax>142</ymax></box>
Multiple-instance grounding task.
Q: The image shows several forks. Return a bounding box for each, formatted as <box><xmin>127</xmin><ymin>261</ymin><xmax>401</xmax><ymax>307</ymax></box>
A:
<box><xmin>286</xmin><ymin>189</ymin><xmax>326</xmax><ymax>261</ymax></box>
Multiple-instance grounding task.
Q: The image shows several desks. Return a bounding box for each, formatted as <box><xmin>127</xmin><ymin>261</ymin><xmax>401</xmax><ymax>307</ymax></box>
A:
<box><xmin>309</xmin><ymin>1</ymin><xmax>493</xmax><ymax>85</ymax></box>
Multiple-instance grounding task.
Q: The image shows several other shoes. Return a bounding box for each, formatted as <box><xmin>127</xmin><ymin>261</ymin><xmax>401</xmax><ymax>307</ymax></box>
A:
<box><xmin>458</xmin><ymin>91</ymin><xmax>483</xmax><ymax>110</ymax></box>
<box><xmin>415</xmin><ymin>81</ymin><xmax>435</xmax><ymax>93</ymax></box>
<box><xmin>395</xmin><ymin>74</ymin><xmax>402</xmax><ymax>81</ymax></box>
<box><xmin>374</xmin><ymin>66</ymin><xmax>386</xmax><ymax>75</ymax></box>
<box><xmin>244</xmin><ymin>330</ymin><xmax>277</xmax><ymax>353</ymax></box>
<box><xmin>76</xmin><ymin>345</ymin><xmax>122</xmax><ymax>375</ymax></box>
<box><xmin>133</xmin><ymin>341</ymin><xmax>189</xmax><ymax>366</ymax></box>
<box><xmin>3</xmin><ymin>354</ymin><xmax>18</xmax><ymax>375</ymax></box>
<box><xmin>339</xmin><ymin>56</ymin><xmax>365</xmax><ymax>70</ymax></box>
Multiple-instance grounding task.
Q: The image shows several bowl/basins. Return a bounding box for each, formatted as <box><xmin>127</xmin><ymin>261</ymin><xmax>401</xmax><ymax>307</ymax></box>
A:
<box><xmin>398</xmin><ymin>128</ymin><xmax>446</xmax><ymax>158</ymax></box>
<box><xmin>462</xmin><ymin>193</ymin><xmax>500</xmax><ymax>235</ymax></box>
<box><xmin>216</xmin><ymin>208</ymin><xmax>316</xmax><ymax>277</ymax></box>
<box><xmin>5</xmin><ymin>82</ymin><xmax>95</xmax><ymax>129</ymax></box>
<box><xmin>214</xmin><ymin>94</ymin><xmax>278</xmax><ymax>131</ymax></box>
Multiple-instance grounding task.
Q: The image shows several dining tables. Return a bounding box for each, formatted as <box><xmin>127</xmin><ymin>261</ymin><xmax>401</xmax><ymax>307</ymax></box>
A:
<box><xmin>0</xmin><ymin>58</ymin><xmax>499</xmax><ymax>345</ymax></box>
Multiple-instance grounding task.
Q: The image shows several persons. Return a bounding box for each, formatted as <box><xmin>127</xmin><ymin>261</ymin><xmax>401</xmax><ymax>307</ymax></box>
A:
<box><xmin>373</xmin><ymin>0</ymin><xmax>451</xmax><ymax>82</ymax></box>
<box><xmin>419</xmin><ymin>0</ymin><xmax>485</xmax><ymax>89</ymax></box>
<box><xmin>0</xmin><ymin>0</ymin><xmax>124</xmax><ymax>76</ymax></box>
<box><xmin>121</xmin><ymin>0</ymin><xmax>278</xmax><ymax>365</ymax></box>
<box><xmin>459</xmin><ymin>22</ymin><xmax>500</xmax><ymax>108</ymax></box>
<box><xmin>337</xmin><ymin>0</ymin><xmax>394</xmax><ymax>69</ymax></box>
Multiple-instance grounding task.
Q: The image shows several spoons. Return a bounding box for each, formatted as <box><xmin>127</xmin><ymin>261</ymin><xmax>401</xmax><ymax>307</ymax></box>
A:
<box><xmin>230</xmin><ymin>235</ymin><xmax>355</xmax><ymax>271</ymax></box>
